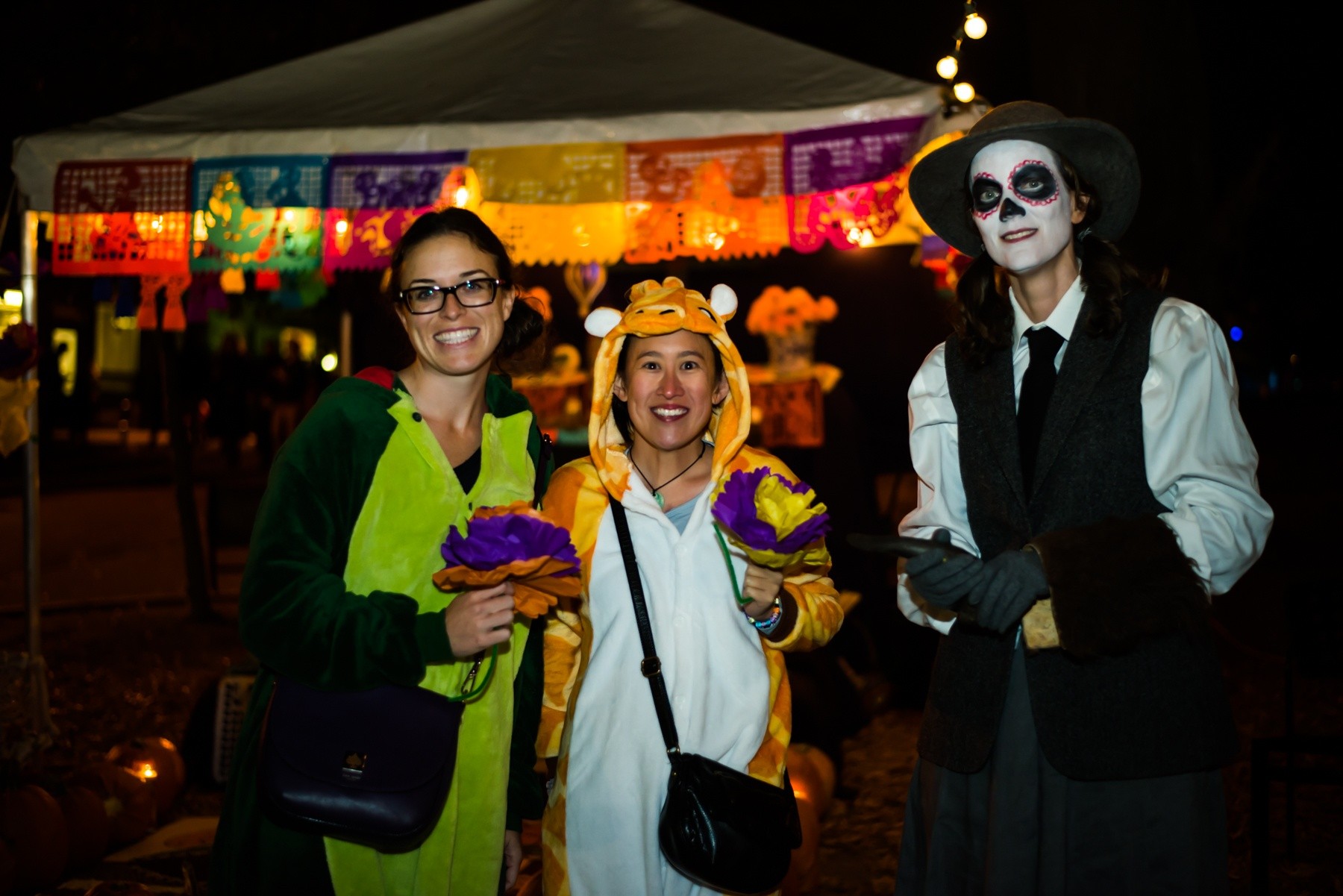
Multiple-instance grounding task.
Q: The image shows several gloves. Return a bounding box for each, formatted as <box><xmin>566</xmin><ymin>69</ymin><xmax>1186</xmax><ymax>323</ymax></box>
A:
<box><xmin>968</xmin><ymin>547</ymin><xmax>1051</xmax><ymax>634</ymax></box>
<box><xmin>905</xmin><ymin>528</ymin><xmax>987</xmax><ymax>611</ymax></box>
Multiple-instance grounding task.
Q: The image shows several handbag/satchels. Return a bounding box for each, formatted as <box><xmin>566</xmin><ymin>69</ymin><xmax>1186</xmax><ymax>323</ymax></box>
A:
<box><xmin>658</xmin><ymin>753</ymin><xmax>803</xmax><ymax>896</ymax></box>
<box><xmin>251</xmin><ymin>668</ymin><xmax>467</xmax><ymax>854</ymax></box>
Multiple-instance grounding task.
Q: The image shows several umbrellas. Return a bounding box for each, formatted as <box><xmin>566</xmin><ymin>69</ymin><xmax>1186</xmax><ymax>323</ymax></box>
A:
<box><xmin>12</xmin><ymin>0</ymin><xmax>956</xmax><ymax>273</ymax></box>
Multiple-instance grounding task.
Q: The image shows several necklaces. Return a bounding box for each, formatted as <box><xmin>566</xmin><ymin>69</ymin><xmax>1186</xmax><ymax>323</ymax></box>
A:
<box><xmin>628</xmin><ymin>440</ymin><xmax>707</xmax><ymax>508</ymax></box>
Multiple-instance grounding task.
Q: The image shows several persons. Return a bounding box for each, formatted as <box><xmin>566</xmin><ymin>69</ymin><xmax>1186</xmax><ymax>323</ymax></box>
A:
<box><xmin>5</xmin><ymin>309</ymin><xmax>588</xmax><ymax>535</ymax></box>
<box><xmin>210</xmin><ymin>203</ymin><xmax>558</xmax><ymax>896</ymax></box>
<box><xmin>531</xmin><ymin>275</ymin><xmax>844</xmax><ymax>896</ymax></box>
<box><xmin>892</xmin><ymin>95</ymin><xmax>1277</xmax><ymax>894</ymax></box>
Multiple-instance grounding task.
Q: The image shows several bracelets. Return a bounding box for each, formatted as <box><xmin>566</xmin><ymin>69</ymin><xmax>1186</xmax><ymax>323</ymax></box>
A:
<box><xmin>745</xmin><ymin>595</ymin><xmax>781</xmax><ymax>628</ymax></box>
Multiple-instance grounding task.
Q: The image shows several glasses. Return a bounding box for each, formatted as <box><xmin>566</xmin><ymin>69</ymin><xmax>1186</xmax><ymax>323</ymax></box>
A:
<box><xmin>396</xmin><ymin>277</ymin><xmax>511</xmax><ymax>316</ymax></box>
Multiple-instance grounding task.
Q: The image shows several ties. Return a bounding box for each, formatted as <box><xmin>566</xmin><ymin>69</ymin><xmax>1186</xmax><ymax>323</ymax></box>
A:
<box><xmin>1014</xmin><ymin>326</ymin><xmax>1066</xmax><ymax>502</ymax></box>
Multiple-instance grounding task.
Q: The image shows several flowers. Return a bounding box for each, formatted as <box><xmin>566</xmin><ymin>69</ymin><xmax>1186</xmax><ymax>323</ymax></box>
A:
<box><xmin>434</xmin><ymin>504</ymin><xmax>588</xmax><ymax>636</ymax></box>
<box><xmin>717</xmin><ymin>467</ymin><xmax>831</xmax><ymax>609</ymax></box>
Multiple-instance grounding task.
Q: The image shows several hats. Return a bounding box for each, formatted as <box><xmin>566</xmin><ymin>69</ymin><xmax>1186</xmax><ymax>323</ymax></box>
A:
<box><xmin>907</xmin><ymin>99</ymin><xmax>1142</xmax><ymax>260</ymax></box>
